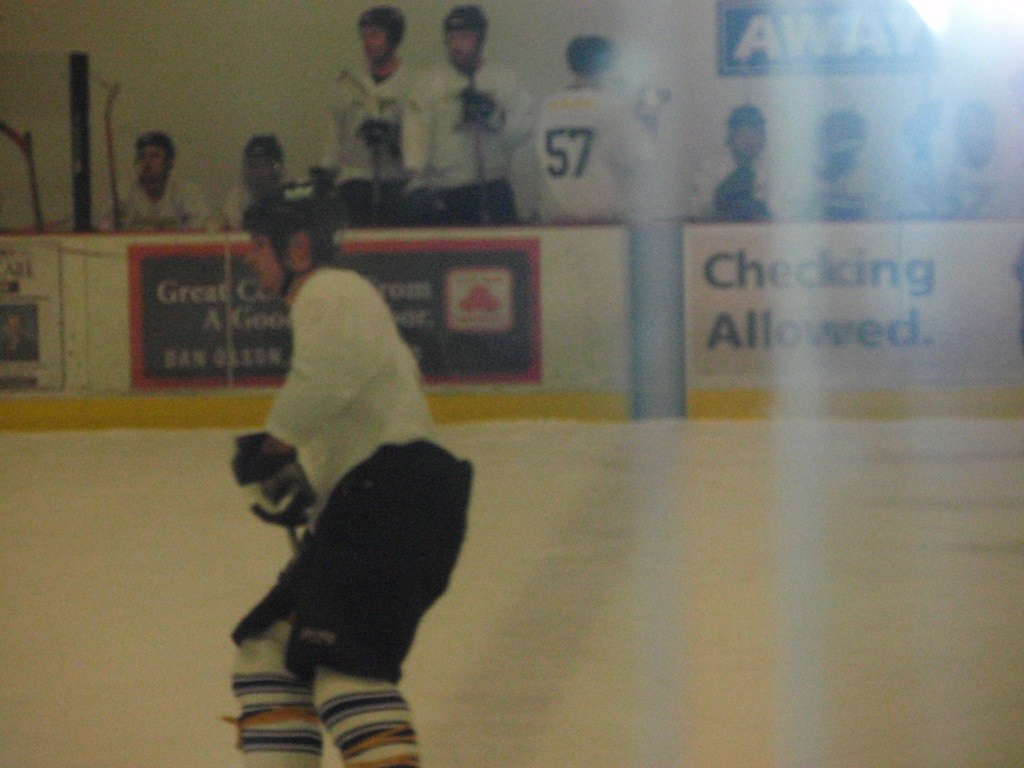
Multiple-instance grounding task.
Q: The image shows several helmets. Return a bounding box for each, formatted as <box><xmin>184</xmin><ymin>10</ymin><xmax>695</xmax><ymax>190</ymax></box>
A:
<box><xmin>133</xmin><ymin>130</ymin><xmax>174</xmax><ymax>164</ymax></box>
<box><xmin>446</xmin><ymin>7</ymin><xmax>487</xmax><ymax>31</ymax></box>
<box><xmin>243</xmin><ymin>179</ymin><xmax>340</xmax><ymax>236</ymax></box>
<box><xmin>241</xmin><ymin>133</ymin><xmax>282</xmax><ymax>168</ymax></box>
<box><xmin>356</xmin><ymin>7</ymin><xmax>402</xmax><ymax>42</ymax></box>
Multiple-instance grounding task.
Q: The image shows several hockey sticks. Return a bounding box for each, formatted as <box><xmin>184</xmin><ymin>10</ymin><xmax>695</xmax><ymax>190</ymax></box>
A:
<box><xmin>106</xmin><ymin>83</ymin><xmax>121</xmax><ymax>234</ymax></box>
<box><xmin>0</xmin><ymin>120</ymin><xmax>44</xmax><ymax>231</ymax></box>
<box><xmin>468</xmin><ymin>67</ymin><xmax>491</xmax><ymax>224</ymax></box>
<box><xmin>372</xmin><ymin>142</ymin><xmax>384</xmax><ymax>230</ymax></box>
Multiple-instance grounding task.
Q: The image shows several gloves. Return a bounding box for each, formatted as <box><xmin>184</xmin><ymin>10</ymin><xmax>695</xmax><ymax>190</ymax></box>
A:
<box><xmin>354</xmin><ymin>118</ymin><xmax>400</xmax><ymax>158</ymax></box>
<box><xmin>252</xmin><ymin>458</ymin><xmax>317</xmax><ymax>527</ymax></box>
<box><xmin>232</xmin><ymin>432</ymin><xmax>295</xmax><ymax>485</ymax></box>
<box><xmin>458</xmin><ymin>87</ymin><xmax>496</xmax><ymax>125</ymax></box>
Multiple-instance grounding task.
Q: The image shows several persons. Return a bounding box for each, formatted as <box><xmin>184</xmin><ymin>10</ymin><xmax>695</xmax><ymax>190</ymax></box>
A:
<box><xmin>536</xmin><ymin>38</ymin><xmax>650</xmax><ymax>226</ymax></box>
<box><xmin>221</xmin><ymin>178</ymin><xmax>472</xmax><ymax>768</ymax></box>
<box><xmin>225</xmin><ymin>136</ymin><xmax>304</xmax><ymax>227</ymax></box>
<box><xmin>312</xmin><ymin>3</ymin><xmax>530</xmax><ymax>231</ymax></box>
<box><xmin>98</xmin><ymin>130</ymin><xmax>208</xmax><ymax>229</ymax></box>
